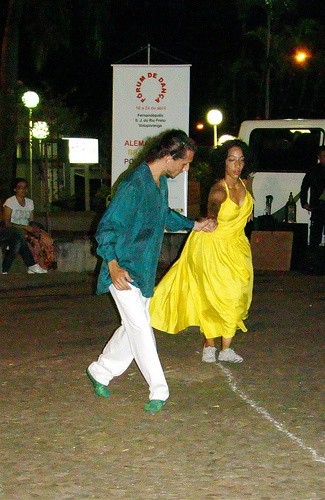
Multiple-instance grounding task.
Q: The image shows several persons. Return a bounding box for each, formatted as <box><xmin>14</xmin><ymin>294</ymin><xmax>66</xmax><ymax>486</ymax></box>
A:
<box><xmin>86</xmin><ymin>129</ymin><xmax>210</xmax><ymax>413</ymax></box>
<box><xmin>0</xmin><ymin>178</ymin><xmax>47</xmax><ymax>274</ymax></box>
<box><xmin>300</xmin><ymin>145</ymin><xmax>325</xmax><ymax>253</ymax></box>
<box><xmin>149</xmin><ymin>138</ymin><xmax>256</xmax><ymax>363</ymax></box>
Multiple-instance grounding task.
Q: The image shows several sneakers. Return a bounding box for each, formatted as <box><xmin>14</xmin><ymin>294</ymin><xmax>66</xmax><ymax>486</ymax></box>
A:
<box><xmin>218</xmin><ymin>347</ymin><xmax>244</xmax><ymax>363</ymax></box>
<box><xmin>202</xmin><ymin>344</ymin><xmax>217</xmax><ymax>363</ymax></box>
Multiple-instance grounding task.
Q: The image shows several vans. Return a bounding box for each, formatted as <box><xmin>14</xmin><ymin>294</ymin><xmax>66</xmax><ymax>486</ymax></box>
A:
<box><xmin>238</xmin><ymin>119</ymin><xmax>325</xmax><ymax>247</ymax></box>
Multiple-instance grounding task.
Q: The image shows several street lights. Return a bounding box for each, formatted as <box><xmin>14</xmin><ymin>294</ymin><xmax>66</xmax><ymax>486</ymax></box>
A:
<box><xmin>206</xmin><ymin>108</ymin><xmax>223</xmax><ymax>149</ymax></box>
<box><xmin>22</xmin><ymin>91</ymin><xmax>49</xmax><ymax>212</ymax></box>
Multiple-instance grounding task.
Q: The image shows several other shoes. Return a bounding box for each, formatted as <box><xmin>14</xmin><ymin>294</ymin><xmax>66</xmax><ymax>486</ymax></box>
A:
<box><xmin>27</xmin><ymin>270</ymin><xmax>34</xmax><ymax>274</ymax></box>
<box><xmin>86</xmin><ymin>367</ymin><xmax>110</xmax><ymax>396</ymax></box>
<box><xmin>144</xmin><ymin>399</ymin><xmax>166</xmax><ymax>413</ymax></box>
<box><xmin>28</xmin><ymin>264</ymin><xmax>48</xmax><ymax>274</ymax></box>
